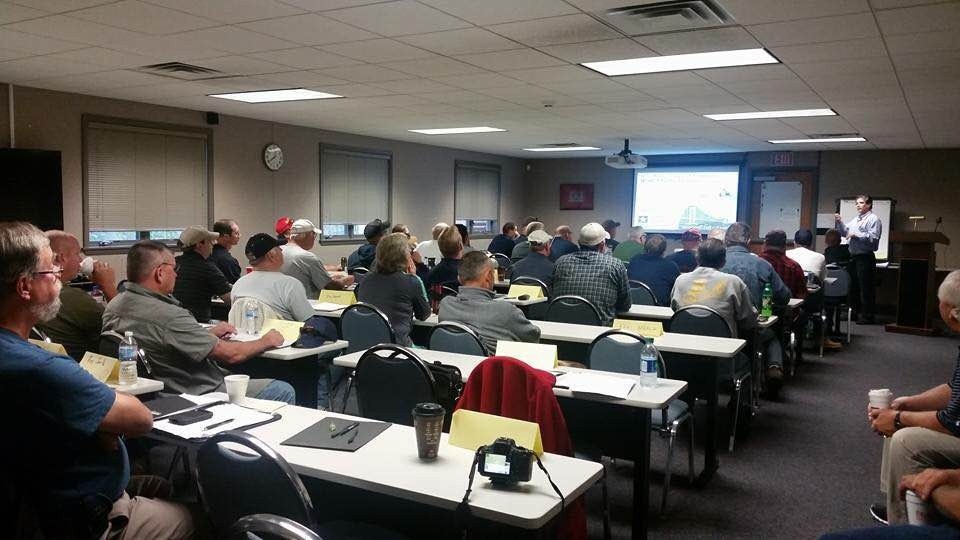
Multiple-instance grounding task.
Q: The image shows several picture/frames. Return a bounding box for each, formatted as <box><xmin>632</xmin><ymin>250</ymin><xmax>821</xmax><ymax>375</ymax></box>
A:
<box><xmin>559</xmin><ymin>183</ymin><xmax>595</xmax><ymax>210</ymax></box>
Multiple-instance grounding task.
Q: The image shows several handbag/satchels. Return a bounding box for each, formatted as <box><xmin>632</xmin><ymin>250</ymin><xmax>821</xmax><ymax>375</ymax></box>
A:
<box><xmin>423</xmin><ymin>362</ymin><xmax>462</xmax><ymax>406</ymax></box>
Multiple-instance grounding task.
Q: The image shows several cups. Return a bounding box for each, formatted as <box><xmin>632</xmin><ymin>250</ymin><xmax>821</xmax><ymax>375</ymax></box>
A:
<box><xmin>411</xmin><ymin>403</ymin><xmax>446</xmax><ymax>459</ymax></box>
<box><xmin>224</xmin><ymin>374</ymin><xmax>249</xmax><ymax>406</ymax></box>
<box><xmin>498</xmin><ymin>266</ymin><xmax>505</xmax><ymax>281</ymax></box>
<box><xmin>906</xmin><ymin>490</ymin><xmax>928</xmax><ymax>525</ymax></box>
<box><xmin>869</xmin><ymin>387</ymin><xmax>893</xmax><ymax>410</ymax></box>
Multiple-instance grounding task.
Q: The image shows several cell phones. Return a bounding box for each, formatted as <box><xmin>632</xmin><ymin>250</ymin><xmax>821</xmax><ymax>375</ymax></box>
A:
<box><xmin>169</xmin><ymin>409</ymin><xmax>213</xmax><ymax>424</ymax></box>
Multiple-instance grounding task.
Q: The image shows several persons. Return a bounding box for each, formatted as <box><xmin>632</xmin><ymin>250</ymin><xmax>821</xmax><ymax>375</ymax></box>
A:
<box><xmin>435</xmin><ymin>250</ymin><xmax>544</xmax><ymax>357</ymax></box>
<box><xmin>392</xmin><ymin>219</ymin><xmax>478</xmax><ymax>288</ymax></box>
<box><xmin>90</xmin><ymin>239</ymin><xmax>299</xmax><ymax>408</ymax></box>
<box><xmin>818</xmin><ymin>464</ymin><xmax>960</xmax><ymax>540</ymax></box>
<box><xmin>353</xmin><ymin>231</ymin><xmax>433</xmax><ymax>350</ymax></box>
<box><xmin>231</xmin><ymin>230</ymin><xmax>340</xmax><ymax>345</ymax></box>
<box><xmin>837</xmin><ymin>192</ymin><xmax>883</xmax><ymax>324</ymax></box>
<box><xmin>167</xmin><ymin>223</ymin><xmax>232</xmax><ymax>323</ymax></box>
<box><xmin>269</xmin><ymin>215</ymin><xmax>295</xmax><ymax>246</ymax></box>
<box><xmin>487</xmin><ymin>215</ymin><xmax>853</xmax><ymax>381</ymax></box>
<box><xmin>35</xmin><ymin>227</ymin><xmax>119</xmax><ymax>358</ymax></box>
<box><xmin>346</xmin><ymin>214</ymin><xmax>391</xmax><ymax>278</ymax></box>
<box><xmin>855</xmin><ymin>267</ymin><xmax>960</xmax><ymax>528</ymax></box>
<box><xmin>274</xmin><ymin>218</ymin><xmax>352</xmax><ymax>295</ymax></box>
<box><xmin>0</xmin><ymin>220</ymin><xmax>199</xmax><ymax>539</ymax></box>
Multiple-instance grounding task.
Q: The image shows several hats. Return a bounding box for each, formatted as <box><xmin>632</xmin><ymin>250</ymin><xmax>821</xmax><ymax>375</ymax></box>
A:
<box><xmin>724</xmin><ymin>222</ymin><xmax>751</xmax><ymax>243</ymax></box>
<box><xmin>179</xmin><ymin>224</ymin><xmax>220</xmax><ymax>248</ymax></box>
<box><xmin>244</xmin><ymin>232</ymin><xmax>288</xmax><ymax>262</ymax></box>
<box><xmin>275</xmin><ymin>216</ymin><xmax>293</xmax><ymax>235</ymax></box>
<box><xmin>364</xmin><ymin>219</ymin><xmax>391</xmax><ymax>240</ymax></box>
<box><xmin>603</xmin><ymin>220</ymin><xmax>621</xmax><ymax>231</ymax></box>
<box><xmin>290</xmin><ymin>219</ymin><xmax>323</xmax><ymax>235</ymax></box>
<box><xmin>555</xmin><ymin>226</ymin><xmax>573</xmax><ymax>237</ymax></box>
<box><xmin>795</xmin><ymin>229</ymin><xmax>814</xmax><ymax>246</ymax></box>
<box><xmin>579</xmin><ymin>222</ymin><xmax>611</xmax><ymax>246</ymax></box>
<box><xmin>766</xmin><ymin>230</ymin><xmax>787</xmax><ymax>247</ymax></box>
<box><xmin>526</xmin><ymin>230</ymin><xmax>554</xmax><ymax>246</ymax></box>
<box><xmin>291</xmin><ymin>316</ymin><xmax>338</xmax><ymax>348</ymax></box>
<box><xmin>679</xmin><ymin>228</ymin><xmax>702</xmax><ymax>240</ymax></box>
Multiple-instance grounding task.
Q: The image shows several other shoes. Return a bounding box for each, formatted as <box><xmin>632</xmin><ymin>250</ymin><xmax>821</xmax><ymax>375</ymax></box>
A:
<box><xmin>838</xmin><ymin>308</ymin><xmax>876</xmax><ymax>325</ymax></box>
<box><xmin>767</xmin><ymin>364</ymin><xmax>788</xmax><ymax>384</ymax></box>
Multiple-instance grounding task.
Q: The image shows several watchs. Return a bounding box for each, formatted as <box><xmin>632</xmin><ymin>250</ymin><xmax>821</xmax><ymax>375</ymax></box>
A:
<box><xmin>892</xmin><ymin>411</ymin><xmax>905</xmax><ymax>432</ymax></box>
<box><xmin>213</xmin><ymin>216</ymin><xmax>243</xmax><ymax>284</ymax></box>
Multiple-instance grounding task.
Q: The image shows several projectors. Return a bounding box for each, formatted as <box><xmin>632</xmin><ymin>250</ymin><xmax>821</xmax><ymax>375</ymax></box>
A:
<box><xmin>605</xmin><ymin>155</ymin><xmax>647</xmax><ymax>169</ymax></box>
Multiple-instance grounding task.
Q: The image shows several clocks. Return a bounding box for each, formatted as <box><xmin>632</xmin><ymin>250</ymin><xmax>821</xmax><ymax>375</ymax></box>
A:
<box><xmin>262</xmin><ymin>142</ymin><xmax>284</xmax><ymax>171</ymax></box>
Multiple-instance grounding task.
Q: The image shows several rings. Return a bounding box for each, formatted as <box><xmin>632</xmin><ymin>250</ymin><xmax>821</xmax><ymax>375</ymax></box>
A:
<box><xmin>883</xmin><ymin>433</ymin><xmax>888</xmax><ymax>439</ymax></box>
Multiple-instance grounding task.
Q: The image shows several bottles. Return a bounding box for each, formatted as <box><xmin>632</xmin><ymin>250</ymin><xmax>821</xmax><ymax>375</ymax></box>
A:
<box><xmin>244</xmin><ymin>297</ymin><xmax>258</xmax><ymax>334</ymax></box>
<box><xmin>761</xmin><ymin>283</ymin><xmax>772</xmax><ymax>317</ymax></box>
<box><xmin>640</xmin><ymin>337</ymin><xmax>657</xmax><ymax>388</ymax></box>
<box><xmin>427</xmin><ymin>258</ymin><xmax>435</xmax><ymax>270</ymax></box>
<box><xmin>118</xmin><ymin>331</ymin><xmax>138</xmax><ymax>385</ymax></box>
<box><xmin>432</xmin><ymin>280</ymin><xmax>442</xmax><ymax>314</ymax></box>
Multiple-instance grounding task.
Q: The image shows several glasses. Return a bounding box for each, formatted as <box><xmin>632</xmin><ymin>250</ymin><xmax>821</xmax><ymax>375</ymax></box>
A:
<box><xmin>159</xmin><ymin>263</ymin><xmax>181</xmax><ymax>272</ymax></box>
<box><xmin>229</xmin><ymin>232</ymin><xmax>240</xmax><ymax>237</ymax></box>
<box><xmin>22</xmin><ymin>266</ymin><xmax>64</xmax><ymax>278</ymax></box>
<box><xmin>404</xmin><ymin>232</ymin><xmax>410</xmax><ymax>239</ymax></box>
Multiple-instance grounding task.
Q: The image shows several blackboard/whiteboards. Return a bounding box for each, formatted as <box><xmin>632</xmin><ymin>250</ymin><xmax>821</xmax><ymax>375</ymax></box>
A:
<box><xmin>839</xmin><ymin>197</ymin><xmax>896</xmax><ymax>263</ymax></box>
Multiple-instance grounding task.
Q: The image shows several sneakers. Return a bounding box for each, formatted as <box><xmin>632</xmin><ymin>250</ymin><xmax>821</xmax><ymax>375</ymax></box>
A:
<box><xmin>869</xmin><ymin>502</ymin><xmax>888</xmax><ymax>525</ymax></box>
<box><xmin>817</xmin><ymin>337</ymin><xmax>842</xmax><ymax>348</ymax></box>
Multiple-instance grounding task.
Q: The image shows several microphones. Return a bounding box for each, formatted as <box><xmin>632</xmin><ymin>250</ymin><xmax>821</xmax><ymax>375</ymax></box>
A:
<box><xmin>934</xmin><ymin>216</ymin><xmax>942</xmax><ymax>232</ymax></box>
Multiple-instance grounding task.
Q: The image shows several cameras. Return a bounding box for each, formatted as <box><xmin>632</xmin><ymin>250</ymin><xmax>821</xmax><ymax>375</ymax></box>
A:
<box><xmin>477</xmin><ymin>436</ymin><xmax>533</xmax><ymax>487</ymax></box>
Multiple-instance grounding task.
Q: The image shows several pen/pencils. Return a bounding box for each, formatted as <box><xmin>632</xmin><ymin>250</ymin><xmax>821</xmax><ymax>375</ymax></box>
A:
<box><xmin>203</xmin><ymin>418</ymin><xmax>235</xmax><ymax>429</ymax></box>
<box><xmin>341</xmin><ymin>422</ymin><xmax>360</xmax><ymax>436</ymax></box>
<box><xmin>331</xmin><ymin>423</ymin><xmax>356</xmax><ymax>438</ymax></box>
<box><xmin>348</xmin><ymin>430</ymin><xmax>359</xmax><ymax>443</ymax></box>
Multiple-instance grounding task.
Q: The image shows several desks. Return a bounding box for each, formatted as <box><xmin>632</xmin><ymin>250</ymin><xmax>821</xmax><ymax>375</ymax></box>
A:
<box><xmin>153</xmin><ymin>272</ymin><xmax>836</xmax><ymax>539</ymax></box>
